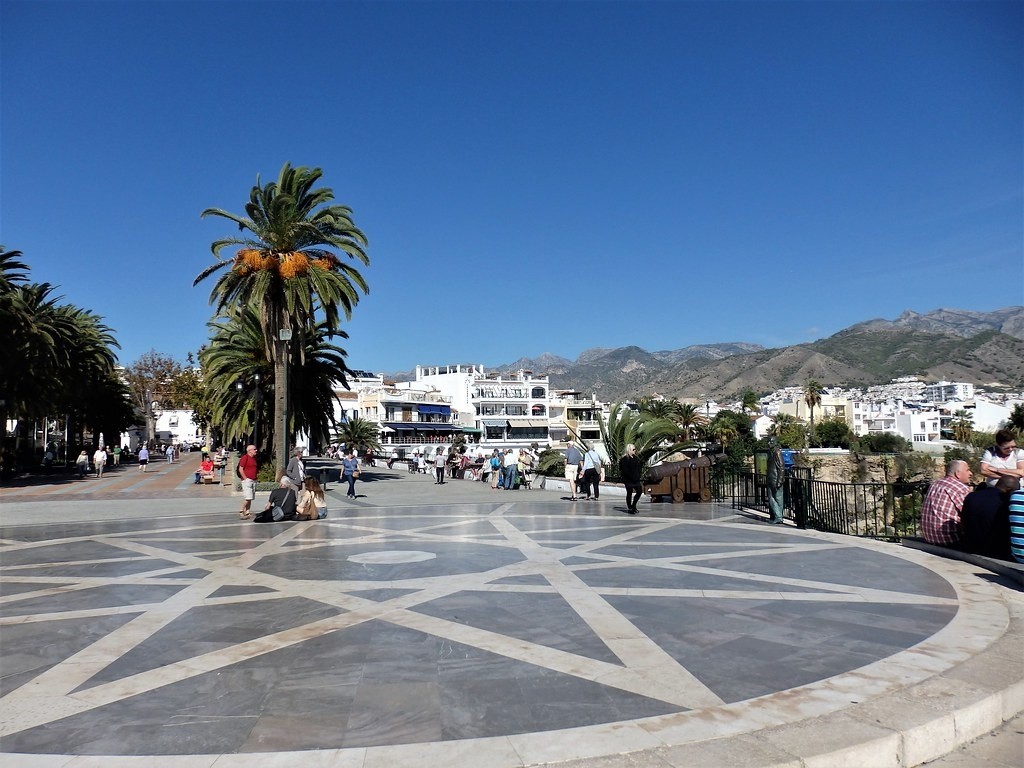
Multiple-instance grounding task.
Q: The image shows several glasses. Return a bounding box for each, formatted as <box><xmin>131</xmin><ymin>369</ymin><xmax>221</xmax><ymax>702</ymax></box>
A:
<box><xmin>255</xmin><ymin>448</ymin><xmax>257</xmax><ymax>450</ymax></box>
<box><xmin>1004</xmin><ymin>445</ymin><xmax>1017</xmax><ymax>450</ymax></box>
<box><xmin>633</xmin><ymin>449</ymin><xmax>636</xmax><ymax>451</ymax></box>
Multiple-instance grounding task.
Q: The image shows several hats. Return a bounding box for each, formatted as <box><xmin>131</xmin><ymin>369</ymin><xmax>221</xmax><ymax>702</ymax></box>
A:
<box><xmin>566</xmin><ymin>441</ymin><xmax>574</xmax><ymax>445</ymax></box>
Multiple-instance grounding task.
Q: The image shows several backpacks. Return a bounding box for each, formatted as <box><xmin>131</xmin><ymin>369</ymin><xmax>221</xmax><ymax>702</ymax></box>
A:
<box><xmin>236</xmin><ymin>455</ymin><xmax>248</xmax><ymax>478</ymax></box>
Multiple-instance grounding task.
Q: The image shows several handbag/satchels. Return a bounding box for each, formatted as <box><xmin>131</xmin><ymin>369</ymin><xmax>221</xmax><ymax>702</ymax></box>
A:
<box><xmin>493</xmin><ymin>458</ymin><xmax>499</xmax><ymax>471</ymax></box>
<box><xmin>272</xmin><ymin>506</ymin><xmax>284</xmax><ymax>521</ymax></box>
<box><xmin>352</xmin><ymin>471</ymin><xmax>360</xmax><ymax>478</ymax></box>
<box><xmin>303</xmin><ymin>499</ymin><xmax>318</xmax><ymax>520</ymax></box>
<box><xmin>594</xmin><ymin>463</ymin><xmax>602</xmax><ymax>474</ymax></box>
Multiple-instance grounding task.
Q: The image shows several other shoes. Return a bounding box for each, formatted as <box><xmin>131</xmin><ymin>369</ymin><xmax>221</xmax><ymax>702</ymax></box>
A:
<box><xmin>585</xmin><ymin>497</ymin><xmax>591</xmax><ymax>500</ymax></box>
<box><xmin>440</xmin><ymin>482</ymin><xmax>443</xmax><ymax>485</ymax></box>
<box><xmin>435</xmin><ymin>482</ymin><xmax>439</xmax><ymax>484</ymax></box>
<box><xmin>347</xmin><ymin>494</ymin><xmax>351</xmax><ymax>500</ymax></box>
<box><xmin>239</xmin><ymin>510</ymin><xmax>248</xmax><ymax>520</ymax></box>
<box><xmin>570</xmin><ymin>496</ymin><xmax>577</xmax><ymax>501</ymax></box>
<box><xmin>352</xmin><ymin>495</ymin><xmax>356</xmax><ymax>501</ymax></box>
<box><xmin>245</xmin><ymin>513</ymin><xmax>252</xmax><ymax>520</ymax></box>
<box><xmin>628</xmin><ymin>507</ymin><xmax>639</xmax><ymax>513</ymax></box>
<box><xmin>591</xmin><ymin>497</ymin><xmax>598</xmax><ymax>500</ymax></box>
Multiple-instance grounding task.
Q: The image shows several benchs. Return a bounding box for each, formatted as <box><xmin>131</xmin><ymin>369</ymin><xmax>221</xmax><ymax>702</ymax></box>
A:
<box><xmin>204</xmin><ymin>456</ymin><xmax>227</xmax><ymax>484</ymax></box>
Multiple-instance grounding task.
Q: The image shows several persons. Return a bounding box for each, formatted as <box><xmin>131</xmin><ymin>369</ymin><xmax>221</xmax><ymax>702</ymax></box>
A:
<box><xmin>77</xmin><ymin>451</ymin><xmax>89</xmax><ymax>479</ymax></box>
<box><xmin>388</xmin><ymin>449</ymin><xmax>400</xmax><ymax>468</ymax></box>
<box><xmin>177</xmin><ymin>442</ymin><xmax>190</xmax><ymax>453</ymax></box>
<box><xmin>239</xmin><ymin>445</ymin><xmax>257</xmax><ymax>519</ymax></box>
<box><xmin>921</xmin><ymin>430</ymin><xmax>1024</xmax><ymax>564</ymax></box>
<box><xmin>367</xmin><ymin>449</ymin><xmax>376</xmax><ymax>467</ymax></box>
<box><xmin>413</xmin><ymin>454</ymin><xmax>428</xmax><ymax>472</ymax></box>
<box><xmin>446</xmin><ymin>448</ymin><xmax>531</xmax><ymax>490</ymax></box>
<box><xmin>767</xmin><ymin>436</ymin><xmax>784</xmax><ymax>523</ymax></box>
<box><xmin>327</xmin><ymin>445</ymin><xmax>362</xmax><ymax>499</ymax></box>
<box><xmin>45</xmin><ymin>450</ymin><xmax>53</xmax><ymax>476</ymax></box>
<box><xmin>93</xmin><ymin>444</ymin><xmax>129</xmax><ymax>478</ymax></box>
<box><xmin>619</xmin><ymin>444</ymin><xmax>643</xmax><ymax>514</ymax></box>
<box><xmin>195</xmin><ymin>438</ymin><xmax>227</xmax><ymax>484</ymax></box>
<box><xmin>266</xmin><ymin>448</ymin><xmax>328</xmax><ymax>519</ymax></box>
<box><xmin>564</xmin><ymin>441</ymin><xmax>601</xmax><ymax>501</ymax></box>
<box><xmin>434</xmin><ymin>450</ymin><xmax>446</xmax><ymax>484</ymax></box>
<box><xmin>136</xmin><ymin>442</ymin><xmax>175</xmax><ymax>472</ymax></box>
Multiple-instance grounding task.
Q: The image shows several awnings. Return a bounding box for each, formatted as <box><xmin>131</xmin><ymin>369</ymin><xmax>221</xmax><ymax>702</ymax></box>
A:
<box><xmin>482</xmin><ymin>420</ymin><xmax>550</xmax><ymax>427</ymax></box>
<box><xmin>385</xmin><ymin>423</ymin><xmax>483</xmax><ymax>433</ymax></box>
